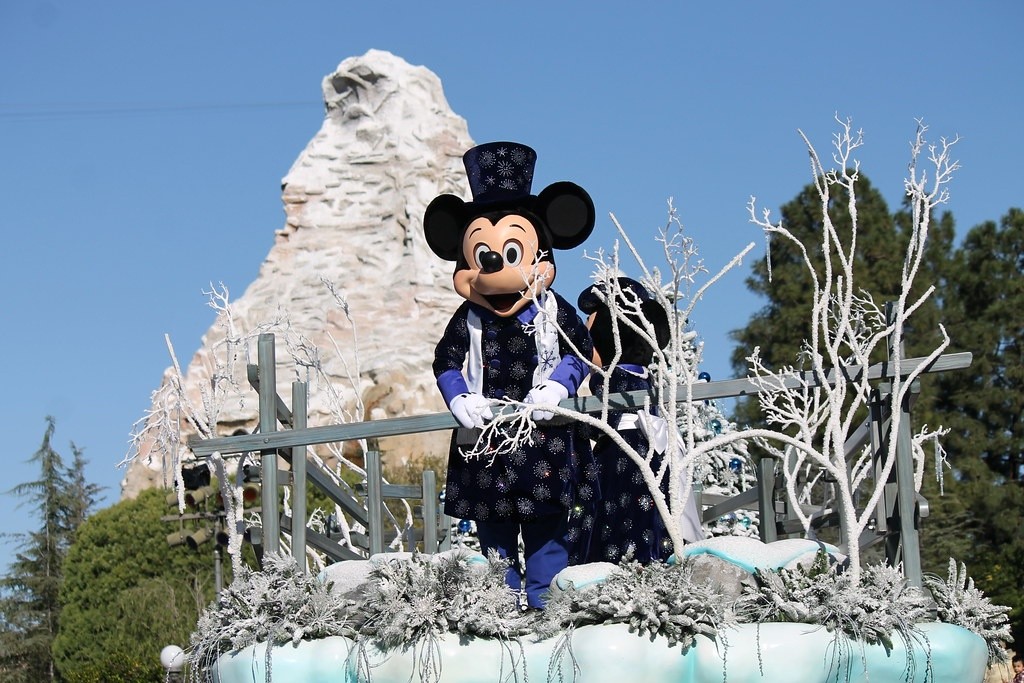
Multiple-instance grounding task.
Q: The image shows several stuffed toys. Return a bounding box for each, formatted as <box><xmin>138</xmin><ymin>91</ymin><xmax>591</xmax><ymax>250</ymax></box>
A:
<box><xmin>578</xmin><ymin>269</ymin><xmax>673</xmax><ymax>568</ymax></box>
<box><xmin>423</xmin><ymin>141</ymin><xmax>594</xmax><ymax>612</ymax></box>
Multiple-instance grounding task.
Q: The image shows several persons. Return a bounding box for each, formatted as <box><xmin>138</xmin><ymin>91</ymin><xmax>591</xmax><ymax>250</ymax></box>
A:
<box><xmin>1012</xmin><ymin>655</ymin><xmax>1024</xmax><ymax>683</ymax></box>
